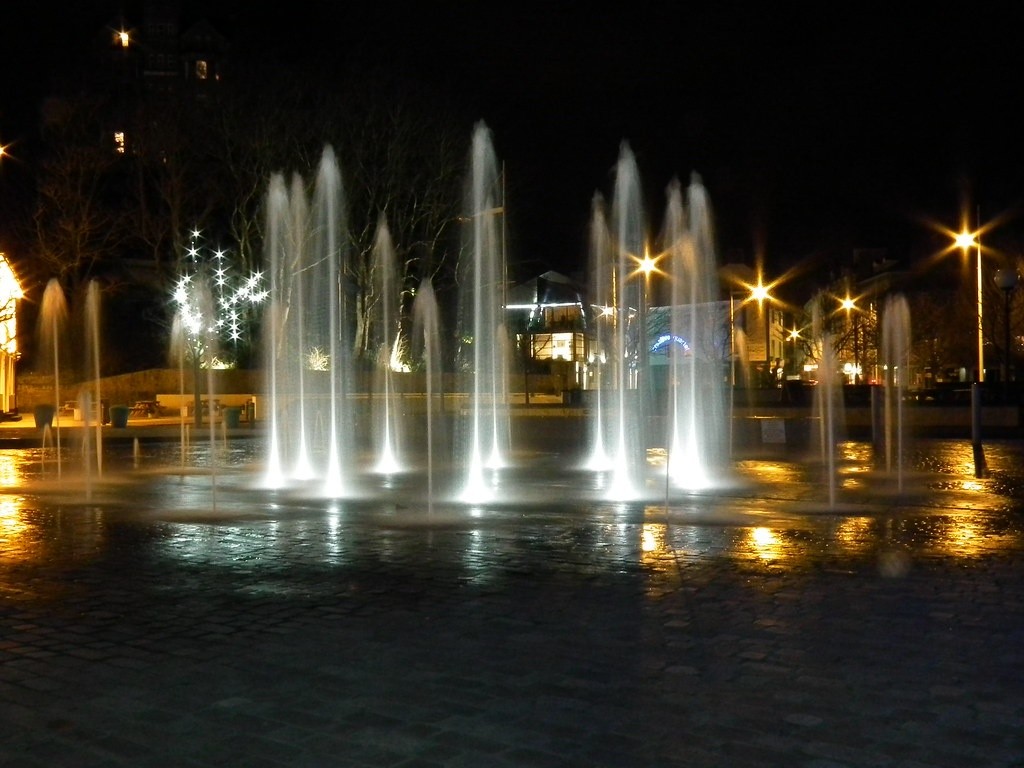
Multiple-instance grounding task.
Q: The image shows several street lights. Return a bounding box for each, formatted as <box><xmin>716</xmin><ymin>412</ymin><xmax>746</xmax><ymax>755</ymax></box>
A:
<box><xmin>994</xmin><ymin>264</ymin><xmax>1018</xmax><ymax>405</ymax></box>
<box><xmin>750</xmin><ymin>282</ymin><xmax>770</xmax><ymax>362</ymax></box>
<box><xmin>636</xmin><ymin>253</ymin><xmax>657</xmax><ymax>451</ymax></box>
<box><xmin>842</xmin><ymin>296</ymin><xmax>858</xmax><ymax>368</ymax></box>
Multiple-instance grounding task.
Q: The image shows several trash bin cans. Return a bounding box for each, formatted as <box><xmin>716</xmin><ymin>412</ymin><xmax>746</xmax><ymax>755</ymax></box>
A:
<box><xmin>223</xmin><ymin>407</ymin><xmax>241</xmax><ymax>428</ymax></box>
<box><xmin>35</xmin><ymin>405</ymin><xmax>53</xmax><ymax>427</ymax></box>
<box><xmin>109</xmin><ymin>405</ymin><xmax>129</xmax><ymax>427</ymax></box>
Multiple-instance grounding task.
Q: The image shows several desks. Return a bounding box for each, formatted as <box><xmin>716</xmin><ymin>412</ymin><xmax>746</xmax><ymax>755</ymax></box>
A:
<box><xmin>135</xmin><ymin>401</ymin><xmax>160</xmax><ymax>416</ymax></box>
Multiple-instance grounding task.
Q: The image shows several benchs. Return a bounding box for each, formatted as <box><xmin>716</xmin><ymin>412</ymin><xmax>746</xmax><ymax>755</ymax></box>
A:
<box><xmin>128</xmin><ymin>406</ymin><xmax>167</xmax><ymax>418</ymax></box>
